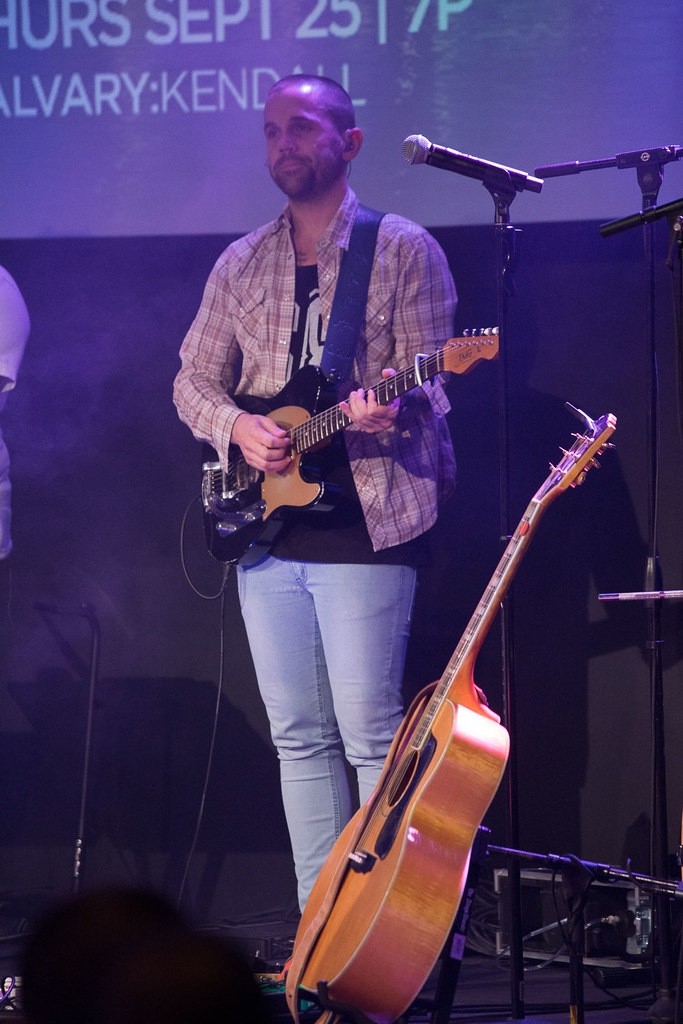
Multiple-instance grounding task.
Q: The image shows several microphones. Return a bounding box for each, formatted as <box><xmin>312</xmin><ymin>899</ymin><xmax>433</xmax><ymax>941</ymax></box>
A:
<box><xmin>402</xmin><ymin>135</ymin><xmax>544</xmax><ymax>195</ymax></box>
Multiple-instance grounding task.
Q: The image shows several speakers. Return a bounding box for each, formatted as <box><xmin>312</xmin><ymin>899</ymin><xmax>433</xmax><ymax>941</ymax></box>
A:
<box><xmin>9</xmin><ymin>675</ymin><xmax>293</xmax><ymax>854</ymax></box>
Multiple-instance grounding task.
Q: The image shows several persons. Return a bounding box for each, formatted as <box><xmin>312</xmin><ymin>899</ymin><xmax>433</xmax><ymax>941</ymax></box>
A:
<box><xmin>0</xmin><ymin>267</ymin><xmax>31</xmax><ymax>560</ymax></box>
<box><xmin>173</xmin><ymin>74</ymin><xmax>461</xmax><ymax>919</ymax></box>
<box><xmin>22</xmin><ymin>886</ymin><xmax>289</xmax><ymax>1024</ymax></box>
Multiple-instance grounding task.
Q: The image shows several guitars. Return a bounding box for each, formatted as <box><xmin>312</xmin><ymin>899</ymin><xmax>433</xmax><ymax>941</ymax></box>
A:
<box><xmin>199</xmin><ymin>327</ymin><xmax>498</xmax><ymax>566</ymax></box>
<box><xmin>280</xmin><ymin>400</ymin><xmax>621</xmax><ymax>1024</ymax></box>
